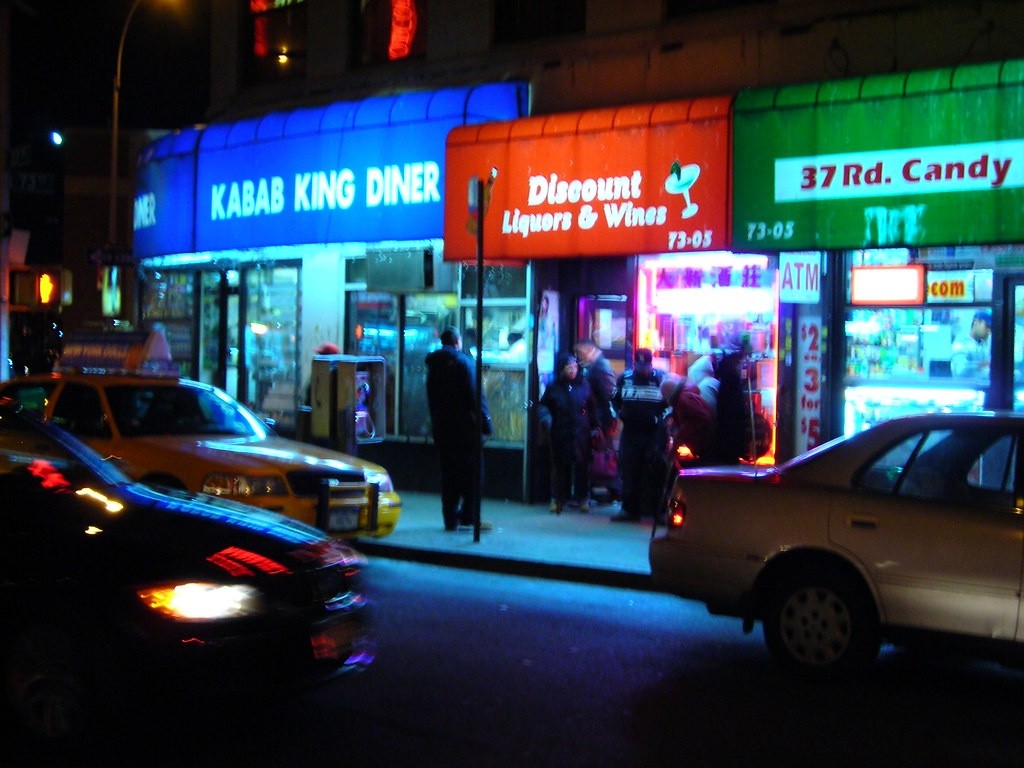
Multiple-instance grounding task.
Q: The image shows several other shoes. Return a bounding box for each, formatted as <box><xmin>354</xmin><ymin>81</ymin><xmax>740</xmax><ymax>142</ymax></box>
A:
<box><xmin>550</xmin><ymin>502</ymin><xmax>562</xmax><ymax>511</ymax></box>
<box><xmin>457</xmin><ymin>521</ymin><xmax>492</xmax><ymax>530</ymax></box>
<box><xmin>611</xmin><ymin>511</ymin><xmax>643</xmax><ymax>522</ymax></box>
<box><xmin>445</xmin><ymin>524</ymin><xmax>456</xmax><ymax>530</ymax></box>
<box><xmin>580</xmin><ymin>501</ymin><xmax>589</xmax><ymax>511</ymax></box>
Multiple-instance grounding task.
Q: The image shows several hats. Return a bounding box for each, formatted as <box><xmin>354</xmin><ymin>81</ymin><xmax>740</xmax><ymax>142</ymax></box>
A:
<box><xmin>440</xmin><ymin>326</ymin><xmax>459</xmax><ymax>344</ymax></box>
<box><xmin>313</xmin><ymin>342</ymin><xmax>340</xmax><ymax>354</ymax></box>
<box><xmin>634</xmin><ymin>348</ymin><xmax>652</xmax><ymax>365</ymax></box>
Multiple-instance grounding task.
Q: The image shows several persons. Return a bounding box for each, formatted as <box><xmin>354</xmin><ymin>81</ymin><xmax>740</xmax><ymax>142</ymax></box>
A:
<box><xmin>507</xmin><ymin>333</ymin><xmax>526</xmax><ymax>351</ymax></box>
<box><xmin>466</xmin><ymin>329</ymin><xmax>477</xmax><ymax>357</ymax></box>
<box><xmin>535</xmin><ymin>341</ymin><xmax>755</xmax><ymax>525</ymax></box>
<box><xmin>425</xmin><ymin>327</ymin><xmax>495</xmax><ymax>531</ymax></box>
<box><xmin>538</xmin><ymin>296</ymin><xmax>557</xmax><ymax>390</ymax></box>
<box><xmin>298</xmin><ymin>346</ymin><xmax>338</xmax><ymax>444</ymax></box>
<box><xmin>949</xmin><ymin>309</ymin><xmax>991</xmax><ymax>377</ymax></box>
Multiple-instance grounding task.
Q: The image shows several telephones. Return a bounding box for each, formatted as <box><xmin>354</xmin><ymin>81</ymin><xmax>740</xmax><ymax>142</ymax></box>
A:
<box><xmin>356</xmin><ymin>374</ymin><xmax>371</xmax><ymax>410</ymax></box>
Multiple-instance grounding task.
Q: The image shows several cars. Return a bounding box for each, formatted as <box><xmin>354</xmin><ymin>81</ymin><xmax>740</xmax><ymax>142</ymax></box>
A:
<box><xmin>0</xmin><ymin>321</ymin><xmax>403</xmax><ymax>550</ymax></box>
<box><xmin>645</xmin><ymin>411</ymin><xmax>1024</xmax><ymax>684</ymax></box>
<box><xmin>0</xmin><ymin>402</ymin><xmax>376</xmax><ymax>737</ymax></box>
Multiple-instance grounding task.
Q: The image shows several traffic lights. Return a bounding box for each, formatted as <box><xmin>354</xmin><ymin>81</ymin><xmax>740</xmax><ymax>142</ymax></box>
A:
<box><xmin>32</xmin><ymin>269</ymin><xmax>73</xmax><ymax>311</ymax></box>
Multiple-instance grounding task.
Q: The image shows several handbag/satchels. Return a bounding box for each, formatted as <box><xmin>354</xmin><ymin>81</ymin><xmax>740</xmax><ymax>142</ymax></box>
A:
<box><xmin>587</xmin><ymin>427</ymin><xmax>619</xmax><ymax>479</ymax></box>
